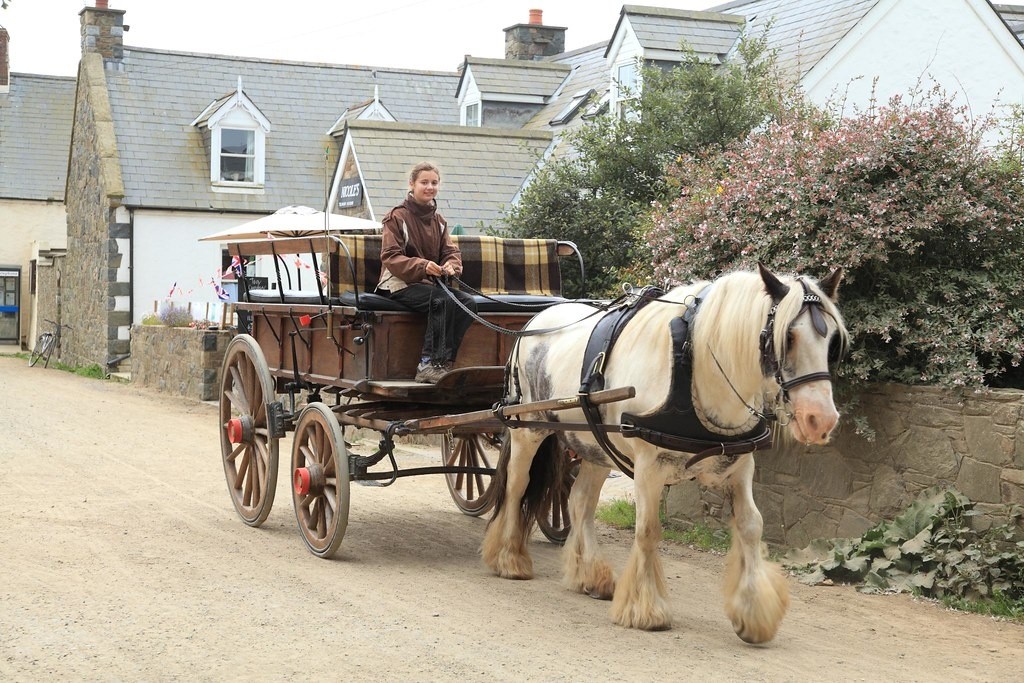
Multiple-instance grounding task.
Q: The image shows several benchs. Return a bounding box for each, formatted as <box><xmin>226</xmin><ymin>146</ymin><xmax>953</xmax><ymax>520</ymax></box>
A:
<box><xmin>328</xmin><ymin>233</ymin><xmax>594</xmax><ymax>314</ymax></box>
<box><xmin>226</xmin><ymin>235</ymin><xmax>338</xmax><ymax>306</ymax></box>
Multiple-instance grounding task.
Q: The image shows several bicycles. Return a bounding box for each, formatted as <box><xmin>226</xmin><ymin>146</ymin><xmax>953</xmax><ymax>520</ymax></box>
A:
<box><xmin>29</xmin><ymin>319</ymin><xmax>73</xmax><ymax>369</ymax></box>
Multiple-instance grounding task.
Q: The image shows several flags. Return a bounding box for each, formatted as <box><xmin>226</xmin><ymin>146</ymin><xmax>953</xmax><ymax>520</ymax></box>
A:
<box><xmin>167</xmin><ymin>255</ymin><xmax>327</xmax><ymax>301</ymax></box>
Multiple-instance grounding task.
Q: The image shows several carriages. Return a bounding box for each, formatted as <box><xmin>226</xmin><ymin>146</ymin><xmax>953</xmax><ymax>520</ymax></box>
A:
<box><xmin>197</xmin><ymin>205</ymin><xmax>849</xmax><ymax>645</ymax></box>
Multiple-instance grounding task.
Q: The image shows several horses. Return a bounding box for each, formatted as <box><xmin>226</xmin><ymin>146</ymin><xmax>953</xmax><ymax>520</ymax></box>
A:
<box><xmin>477</xmin><ymin>259</ymin><xmax>850</xmax><ymax>645</ymax></box>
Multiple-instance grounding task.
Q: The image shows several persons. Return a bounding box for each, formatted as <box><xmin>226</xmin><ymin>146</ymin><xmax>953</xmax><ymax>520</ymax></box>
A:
<box><xmin>374</xmin><ymin>161</ymin><xmax>477</xmax><ymax>383</ymax></box>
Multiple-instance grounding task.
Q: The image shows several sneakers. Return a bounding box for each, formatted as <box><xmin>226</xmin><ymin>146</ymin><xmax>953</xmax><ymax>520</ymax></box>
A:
<box><xmin>414</xmin><ymin>359</ymin><xmax>450</xmax><ymax>383</ymax></box>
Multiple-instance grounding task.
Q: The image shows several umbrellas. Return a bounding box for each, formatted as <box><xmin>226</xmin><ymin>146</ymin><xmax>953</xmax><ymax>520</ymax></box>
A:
<box><xmin>198</xmin><ymin>204</ymin><xmax>385</xmax><ymax>291</ymax></box>
